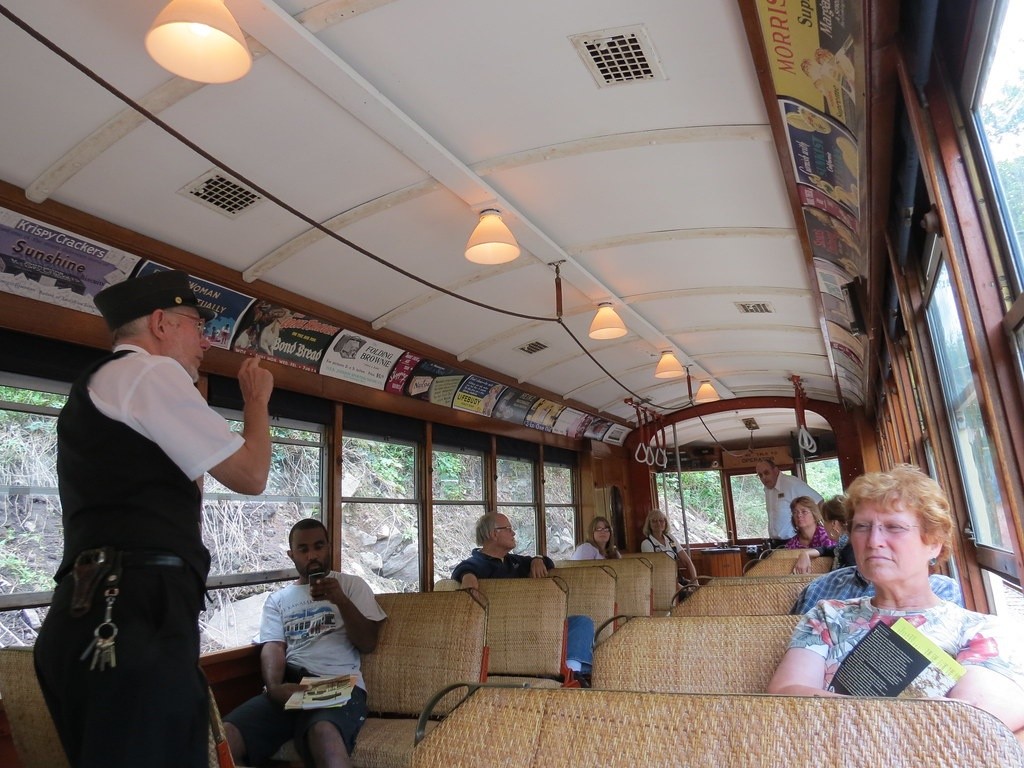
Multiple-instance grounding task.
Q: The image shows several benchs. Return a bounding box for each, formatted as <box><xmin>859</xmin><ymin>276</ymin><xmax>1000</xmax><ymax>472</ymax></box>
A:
<box><xmin>0</xmin><ymin>544</ymin><xmax>1024</xmax><ymax>768</ymax></box>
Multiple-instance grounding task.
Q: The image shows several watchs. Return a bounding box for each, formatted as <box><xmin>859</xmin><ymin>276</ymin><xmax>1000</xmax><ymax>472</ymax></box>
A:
<box><xmin>531</xmin><ymin>555</ymin><xmax>543</xmax><ymax>560</ymax></box>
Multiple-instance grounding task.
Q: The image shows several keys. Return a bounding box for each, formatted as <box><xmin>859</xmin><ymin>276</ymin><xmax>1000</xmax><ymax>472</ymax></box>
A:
<box><xmin>81</xmin><ymin>637</ymin><xmax>116</xmax><ymax>671</ymax></box>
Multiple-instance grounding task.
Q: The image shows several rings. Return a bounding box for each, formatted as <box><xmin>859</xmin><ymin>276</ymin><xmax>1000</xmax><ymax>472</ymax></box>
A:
<box><xmin>316</xmin><ymin>579</ymin><xmax>320</xmax><ymax>585</ymax></box>
<box><xmin>325</xmin><ymin>593</ymin><xmax>327</xmax><ymax>596</ymax></box>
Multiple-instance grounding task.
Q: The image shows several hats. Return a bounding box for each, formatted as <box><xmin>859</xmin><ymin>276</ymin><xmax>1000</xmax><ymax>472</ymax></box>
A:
<box><xmin>93</xmin><ymin>270</ymin><xmax>218</xmax><ymax>331</ymax></box>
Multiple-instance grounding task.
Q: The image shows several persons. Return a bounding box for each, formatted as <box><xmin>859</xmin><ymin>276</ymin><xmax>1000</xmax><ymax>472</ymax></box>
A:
<box><xmin>791</xmin><ymin>494</ymin><xmax>858</xmax><ymax>574</ymax></box>
<box><xmin>640</xmin><ymin>510</ymin><xmax>699</xmax><ymax>592</ymax></box>
<box><xmin>788</xmin><ymin>565</ymin><xmax>966</xmax><ymax>614</ymax></box>
<box><xmin>764</xmin><ymin>463</ymin><xmax>1024</xmax><ymax>733</ymax></box>
<box><xmin>756</xmin><ymin>459</ymin><xmax>825</xmax><ymax>548</ymax></box>
<box><xmin>34</xmin><ymin>269</ymin><xmax>274</xmax><ymax>768</ymax></box>
<box><xmin>569</xmin><ymin>517</ymin><xmax>623</xmax><ymax>560</ymax></box>
<box><xmin>783</xmin><ymin>496</ymin><xmax>837</xmax><ymax>549</ymax></box>
<box><xmin>451</xmin><ymin>511</ymin><xmax>554</xmax><ymax>589</ymax></box>
<box><xmin>220</xmin><ymin>519</ymin><xmax>387</xmax><ymax>768</ymax></box>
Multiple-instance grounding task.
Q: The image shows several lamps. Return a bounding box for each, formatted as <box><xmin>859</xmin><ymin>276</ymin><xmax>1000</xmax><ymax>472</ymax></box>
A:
<box><xmin>589</xmin><ymin>302</ymin><xmax>628</xmax><ymax>339</ymax></box>
<box><xmin>144</xmin><ymin>0</ymin><xmax>252</xmax><ymax>84</ymax></box>
<box><xmin>464</xmin><ymin>209</ymin><xmax>521</xmax><ymax>264</ymax></box>
<box><xmin>655</xmin><ymin>351</ymin><xmax>684</xmax><ymax>378</ymax></box>
<box><xmin>695</xmin><ymin>381</ymin><xmax>720</xmax><ymax>403</ymax></box>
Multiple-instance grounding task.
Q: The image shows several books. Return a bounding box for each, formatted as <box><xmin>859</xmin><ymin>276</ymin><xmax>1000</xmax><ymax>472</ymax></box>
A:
<box><xmin>284</xmin><ymin>673</ymin><xmax>359</xmax><ymax>710</ymax></box>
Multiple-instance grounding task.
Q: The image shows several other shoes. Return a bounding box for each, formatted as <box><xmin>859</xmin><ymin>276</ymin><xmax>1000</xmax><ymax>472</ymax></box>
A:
<box><xmin>550</xmin><ymin>671</ymin><xmax>589</xmax><ymax>688</ymax></box>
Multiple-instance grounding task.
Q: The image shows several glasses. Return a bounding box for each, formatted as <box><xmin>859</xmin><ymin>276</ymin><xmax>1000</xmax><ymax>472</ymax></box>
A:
<box><xmin>495</xmin><ymin>526</ymin><xmax>512</xmax><ymax>533</ymax></box>
<box><xmin>792</xmin><ymin>509</ymin><xmax>810</xmax><ymax>515</ymax></box>
<box><xmin>163</xmin><ymin>310</ymin><xmax>205</xmax><ymax>334</ymax></box>
<box><xmin>756</xmin><ymin>471</ymin><xmax>771</xmax><ymax>478</ymax></box>
<box><xmin>843</xmin><ymin>520</ymin><xmax>919</xmax><ymax>539</ymax></box>
<box><xmin>596</xmin><ymin>527</ymin><xmax>610</xmax><ymax>532</ymax></box>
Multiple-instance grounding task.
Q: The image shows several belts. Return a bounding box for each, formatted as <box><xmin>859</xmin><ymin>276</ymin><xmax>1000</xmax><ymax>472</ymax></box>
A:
<box><xmin>62</xmin><ymin>554</ymin><xmax>192</xmax><ymax>568</ymax></box>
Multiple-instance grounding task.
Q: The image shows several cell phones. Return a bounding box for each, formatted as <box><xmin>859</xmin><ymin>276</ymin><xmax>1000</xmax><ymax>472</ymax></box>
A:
<box><xmin>309</xmin><ymin>572</ymin><xmax>325</xmax><ymax>587</ymax></box>
